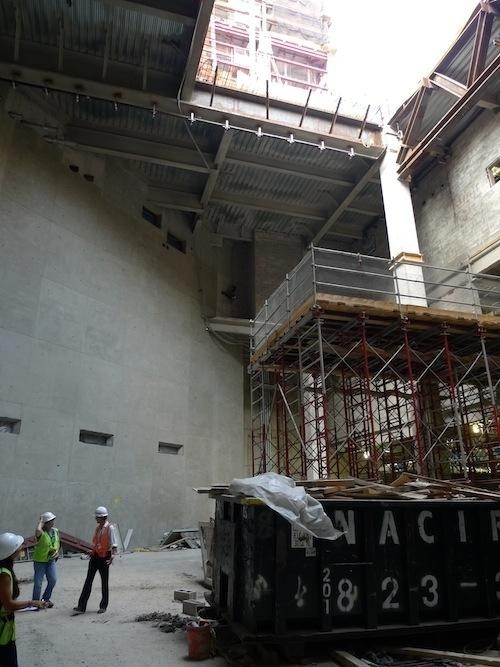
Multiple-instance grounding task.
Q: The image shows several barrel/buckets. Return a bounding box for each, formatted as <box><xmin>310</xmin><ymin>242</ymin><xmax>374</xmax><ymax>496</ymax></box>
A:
<box><xmin>187</xmin><ymin>620</ymin><xmax>211</xmax><ymax>658</ymax></box>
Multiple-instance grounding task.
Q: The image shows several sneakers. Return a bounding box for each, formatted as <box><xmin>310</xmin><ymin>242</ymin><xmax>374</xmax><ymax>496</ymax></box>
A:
<box><xmin>96</xmin><ymin>608</ymin><xmax>107</xmax><ymax>614</ymax></box>
<box><xmin>72</xmin><ymin>607</ymin><xmax>86</xmax><ymax>614</ymax></box>
<box><xmin>41</xmin><ymin>599</ymin><xmax>54</xmax><ymax>609</ymax></box>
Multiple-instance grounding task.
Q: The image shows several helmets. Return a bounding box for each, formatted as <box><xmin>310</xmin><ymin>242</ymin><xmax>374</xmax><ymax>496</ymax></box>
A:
<box><xmin>95</xmin><ymin>506</ymin><xmax>109</xmax><ymax>518</ymax></box>
<box><xmin>0</xmin><ymin>532</ymin><xmax>25</xmax><ymax>562</ymax></box>
<box><xmin>40</xmin><ymin>512</ymin><xmax>57</xmax><ymax>524</ymax></box>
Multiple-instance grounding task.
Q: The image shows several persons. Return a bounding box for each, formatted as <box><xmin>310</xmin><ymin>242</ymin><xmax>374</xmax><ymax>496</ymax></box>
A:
<box><xmin>1</xmin><ymin>531</ymin><xmax>49</xmax><ymax>667</ymax></box>
<box><xmin>72</xmin><ymin>506</ymin><xmax>119</xmax><ymax>614</ymax></box>
<box><xmin>31</xmin><ymin>511</ymin><xmax>62</xmax><ymax>608</ymax></box>
